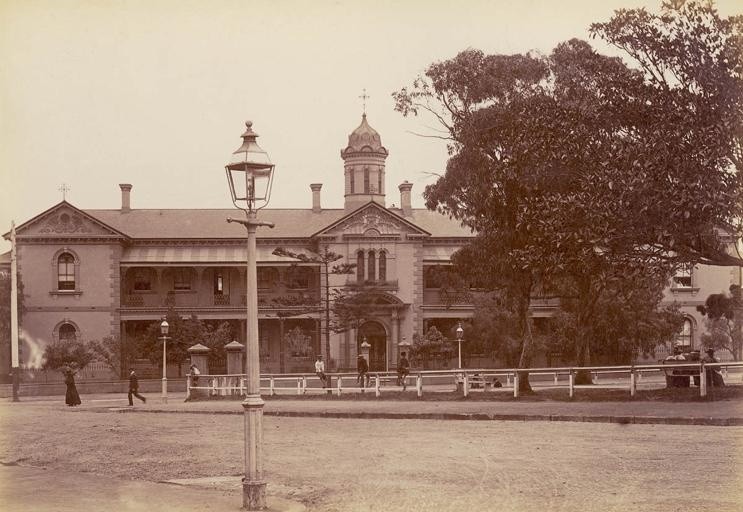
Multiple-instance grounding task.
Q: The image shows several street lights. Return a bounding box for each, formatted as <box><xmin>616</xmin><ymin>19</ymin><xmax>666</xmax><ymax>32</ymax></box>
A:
<box><xmin>158</xmin><ymin>315</ymin><xmax>172</xmax><ymax>405</ymax></box>
<box><xmin>224</xmin><ymin>121</ymin><xmax>275</xmax><ymax>512</ymax></box>
<box><xmin>454</xmin><ymin>323</ymin><xmax>465</xmax><ymax>369</ymax></box>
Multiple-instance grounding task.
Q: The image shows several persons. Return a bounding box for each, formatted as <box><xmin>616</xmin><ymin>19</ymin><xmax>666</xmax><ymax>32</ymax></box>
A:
<box><xmin>352</xmin><ymin>355</ymin><xmax>372</xmax><ymax>387</ymax></box>
<box><xmin>470</xmin><ymin>373</ymin><xmax>480</xmax><ymax>389</ymax></box>
<box><xmin>700</xmin><ymin>347</ymin><xmax>726</xmax><ymax>388</ymax></box>
<box><xmin>492</xmin><ymin>377</ymin><xmax>502</xmax><ymax>388</ymax></box>
<box><xmin>188</xmin><ymin>364</ymin><xmax>200</xmax><ymax>401</ymax></box>
<box><xmin>63</xmin><ymin>368</ymin><xmax>81</xmax><ymax>406</ymax></box>
<box><xmin>124</xmin><ymin>368</ymin><xmax>146</xmax><ymax>407</ymax></box>
<box><xmin>664</xmin><ymin>346</ymin><xmax>687</xmax><ymax>362</ymax></box>
<box><xmin>396</xmin><ymin>350</ymin><xmax>410</xmax><ymax>391</ymax></box>
<box><xmin>313</xmin><ymin>357</ymin><xmax>328</xmax><ymax>386</ymax></box>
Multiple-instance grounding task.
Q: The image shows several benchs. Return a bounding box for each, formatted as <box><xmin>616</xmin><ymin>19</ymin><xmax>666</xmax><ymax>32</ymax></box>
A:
<box><xmin>453</xmin><ymin>368</ymin><xmax>495</xmax><ymax>391</ymax></box>
<box><xmin>658</xmin><ymin>358</ymin><xmax>718</xmax><ymax>386</ymax></box>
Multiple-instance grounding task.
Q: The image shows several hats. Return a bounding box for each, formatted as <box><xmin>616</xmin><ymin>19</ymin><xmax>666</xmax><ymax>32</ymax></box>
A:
<box><xmin>316</xmin><ymin>355</ymin><xmax>322</xmax><ymax>359</ymax></box>
<box><xmin>356</xmin><ymin>356</ymin><xmax>363</xmax><ymax>359</ymax></box>
